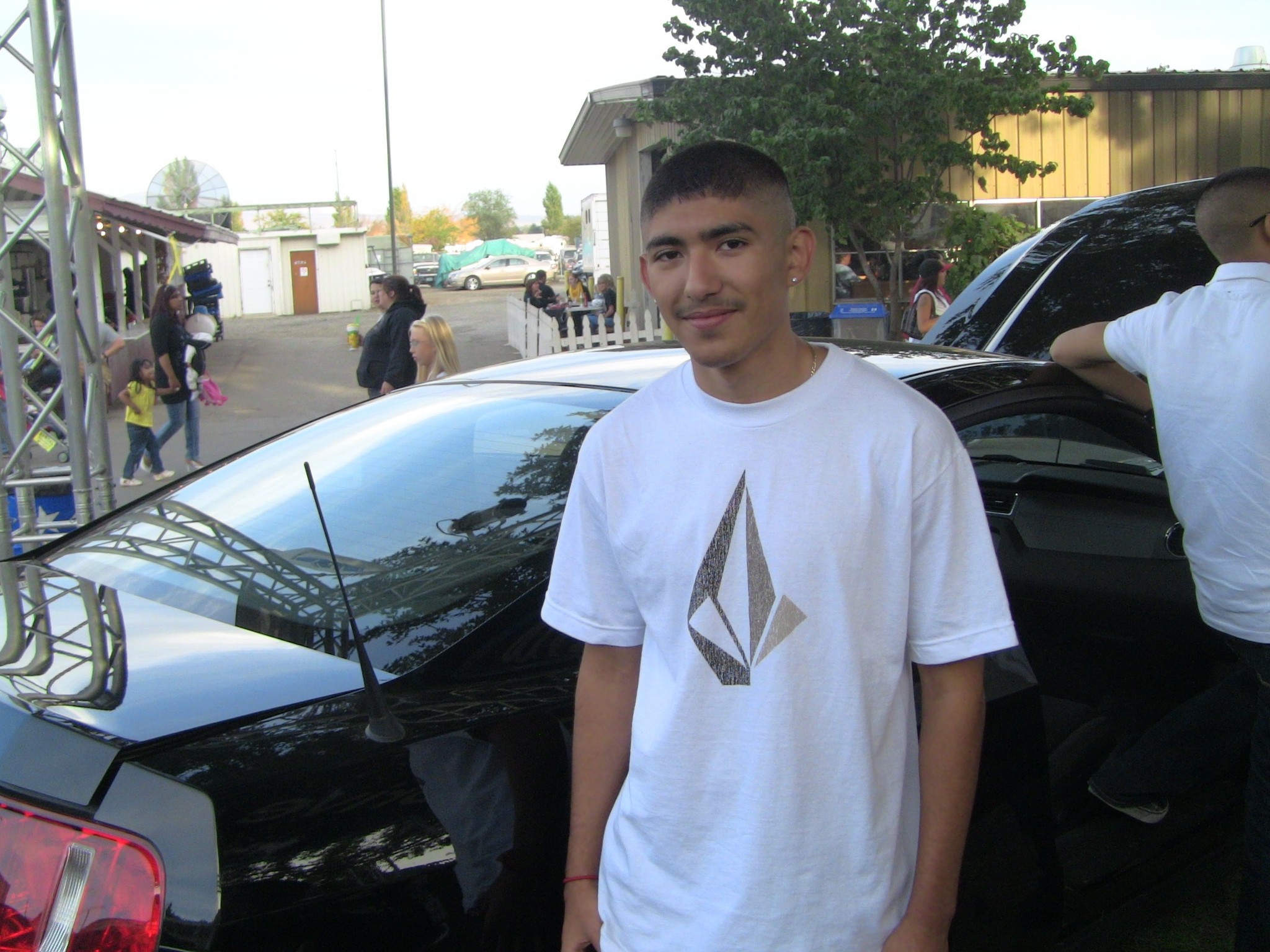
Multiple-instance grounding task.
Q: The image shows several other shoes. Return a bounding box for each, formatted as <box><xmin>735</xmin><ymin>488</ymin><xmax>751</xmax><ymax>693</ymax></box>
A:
<box><xmin>3</xmin><ymin>452</ymin><xmax>10</xmax><ymax>457</ymax></box>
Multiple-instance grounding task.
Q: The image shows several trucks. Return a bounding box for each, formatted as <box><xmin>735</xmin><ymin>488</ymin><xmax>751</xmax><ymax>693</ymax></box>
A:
<box><xmin>412</xmin><ymin>249</ymin><xmax>439</xmax><ymax>284</ymax></box>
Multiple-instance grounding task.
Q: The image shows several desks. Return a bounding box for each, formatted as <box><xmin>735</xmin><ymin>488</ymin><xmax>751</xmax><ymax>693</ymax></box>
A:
<box><xmin>99</xmin><ymin>319</ymin><xmax>151</xmax><ymax>406</ymax></box>
<box><xmin>564</xmin><ymin>306</ymin><xmax>604</xmax><ymax>336</ymax></box>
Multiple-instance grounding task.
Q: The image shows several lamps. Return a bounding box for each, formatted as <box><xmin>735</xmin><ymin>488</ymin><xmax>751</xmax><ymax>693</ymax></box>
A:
<box><xmin>613</xmin><ymin>113</ymin><xmax>634</xmax><ymax>137</ymax></box>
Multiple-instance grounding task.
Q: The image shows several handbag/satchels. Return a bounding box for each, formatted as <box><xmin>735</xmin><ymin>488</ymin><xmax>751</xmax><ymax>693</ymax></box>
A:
<box><xmin>899</xmin><ymin>292</ymin><xmax>939</xmax><ymax>340</ymax></box>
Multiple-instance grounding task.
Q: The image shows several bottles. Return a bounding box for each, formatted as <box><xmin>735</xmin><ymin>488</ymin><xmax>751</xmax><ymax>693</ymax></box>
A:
<box><xmin>579</xmin><ymin>292</ymin><xmax>584</xmax><ymax>306</ymax></box>
<box><xmin>583</xmin><ymin>292</ymin><xmax>587</xmax><ymax>306</ymax></box>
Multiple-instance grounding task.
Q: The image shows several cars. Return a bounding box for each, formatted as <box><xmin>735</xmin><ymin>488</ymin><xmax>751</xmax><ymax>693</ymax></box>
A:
<box><xmin>531</xmin><ymin>252</ymin><xmax>586</xmax><ymax>284</ymax></box>
<box><xmin>0</xmin><ymin>337</ymin><xmax>1270</xmax><ymax>952</ymax></box>
<box><xmin>446</xmin><ymin>255</ymin><xmax>557</xmax><ymax>290</ymax></box>
<box><xmin>919</xmin><ymin>176</ymin><xmax>1218</xmax><ymax>361</ymax></box>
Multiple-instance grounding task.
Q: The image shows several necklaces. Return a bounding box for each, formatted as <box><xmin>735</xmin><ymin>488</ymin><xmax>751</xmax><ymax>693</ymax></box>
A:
<box><xmin>805</xmin><ymin>340</ymin><xmax>817</xmax><ymax>377</ymax></box>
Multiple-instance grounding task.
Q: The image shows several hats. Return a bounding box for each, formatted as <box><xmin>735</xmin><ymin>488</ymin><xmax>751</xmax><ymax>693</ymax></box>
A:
<box><xmin>918</xmin><ymin>259</ymin><xmax>953</xmax><ymax>281</ymax></box>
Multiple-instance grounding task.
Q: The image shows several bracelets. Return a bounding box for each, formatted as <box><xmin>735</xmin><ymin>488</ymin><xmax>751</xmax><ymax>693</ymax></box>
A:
<box><xmin>559</xmin><ymin>875</ymin><xmax>599</xmax><ymax>897</ymax></box>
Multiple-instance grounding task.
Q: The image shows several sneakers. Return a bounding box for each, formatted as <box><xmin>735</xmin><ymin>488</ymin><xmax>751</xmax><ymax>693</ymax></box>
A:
<box><xmin>155</xmin><ymin>470</ymin><xmax>175</xmax><ymax>481</ymax></box>
<box><xmin>119</xmin><ymin>477</ymin><xmax>143</xmax><ymax>486</ymax></box>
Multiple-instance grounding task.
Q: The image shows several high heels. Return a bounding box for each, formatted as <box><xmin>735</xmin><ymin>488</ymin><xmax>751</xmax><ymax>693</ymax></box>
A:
<box><xmin>186</xmin><ymin>458</ymin><xmax>204</xmax><ymax>470</ymax></box>
<box><xmin>139</xmin><ymin>457</ymin><xmax>153</xmax><ymax>473</ymax></box>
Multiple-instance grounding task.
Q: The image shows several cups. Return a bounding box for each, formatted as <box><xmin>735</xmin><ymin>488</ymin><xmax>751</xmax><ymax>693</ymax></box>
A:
<box><xmin>346</xmin><ymin>324</ymin><xmax>360</xmax><ymax>352</ymax></box>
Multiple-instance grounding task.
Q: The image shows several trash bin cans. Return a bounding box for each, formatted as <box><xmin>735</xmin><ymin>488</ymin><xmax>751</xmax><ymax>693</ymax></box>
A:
<box><xmin>829</xmin><ymin>302</ymin><xmax>890</xmax><ymax>341</ymax></box>
<box><xmin>7</xmin><ymin>467</ymin><xmax>76</xmax><ymax>556</ymax></box>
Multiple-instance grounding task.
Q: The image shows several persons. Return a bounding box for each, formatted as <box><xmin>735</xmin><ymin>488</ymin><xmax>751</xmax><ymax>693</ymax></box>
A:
<box><xmin>903</xmin><ymin>251</ymin><xmax>954</xmax><ymax>344</ymax></box>
<box><xmin>0</xmin><ymin>267</ymin><xmax>215</xmax><ymax>487</ymax></box>
<box><xmin>524</xmin><ymin>270</ymin><xmax>617</xmax><ymax>336</ymax></box>
<box><xmin>540</xmin><ymin>139</ymin><xmax>1020</xmax><ymax>952</ymax></box>
<box><xmin>345</xmin><ymin>275</ymin><xmax>460</xmax><ymax>400</ymax></box>
<box><xmin>834</xmin><ymin>248</ymin><xmax>867</xmax><ymax>299</ymax></box>
<box><xmin>1048</xmin><ymin>165</ymin><xmax>1270</xmax><ymax>952</ymax></box>
